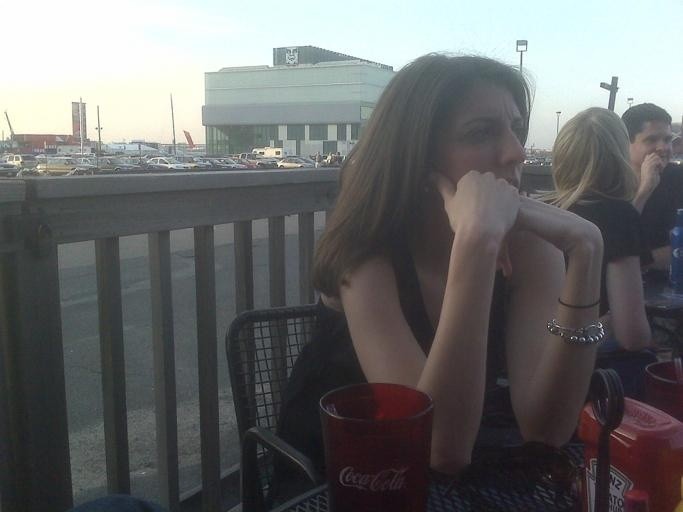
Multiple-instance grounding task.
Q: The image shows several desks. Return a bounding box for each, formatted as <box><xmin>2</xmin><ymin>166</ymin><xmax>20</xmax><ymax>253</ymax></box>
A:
<box><xmin>517</xmin><ymin>40</ymin><xmax>528</xmax><ymax>73</ymax></box>
<box><xmin>556</xmin><ymin>111</ymin><xmax>561</xmax><ymax>135</ymax></box>
<box><xmin>628</xmin><ymin>98</ymin><xmax>633</xmax><ymax>107</ymax></box>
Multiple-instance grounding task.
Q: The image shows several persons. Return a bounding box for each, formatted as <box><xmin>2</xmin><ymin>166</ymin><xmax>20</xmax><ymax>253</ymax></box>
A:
<box><xmin>529</xmin><ymin>106</ymin><xmax>661</xmax><ymax>402</ymax></box>
<box><xmin>621</xmin><ymin>101</ymin><xmax>681</xmax><ymax>276</ymax></box>
<box><xmin>260</xmin><ymin>49</ymin><xmax>608</xmax><ymax>510</ymax></box>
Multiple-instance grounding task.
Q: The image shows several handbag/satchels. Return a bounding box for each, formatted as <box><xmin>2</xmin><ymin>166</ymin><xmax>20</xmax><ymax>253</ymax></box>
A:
<box><xmin>550</xmin><ymin>316</ymin><xmax>604</xmax><ymax>334</ymax></box>
<box><xmin>545</xmin><ymin>320</ymin><xmax>603</xmax><ymax>345</ymax></box>
<box><xmin>555</xmin><ymin>296</ymin><xmax>601</xmax><ymax>311</ymax></box>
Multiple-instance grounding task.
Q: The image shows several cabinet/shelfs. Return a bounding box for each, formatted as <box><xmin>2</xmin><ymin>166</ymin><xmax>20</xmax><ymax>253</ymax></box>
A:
<box><xmin>667</xmin><ymin>209</ymin><xmax>682</xmax><ymax>291</ymax></box>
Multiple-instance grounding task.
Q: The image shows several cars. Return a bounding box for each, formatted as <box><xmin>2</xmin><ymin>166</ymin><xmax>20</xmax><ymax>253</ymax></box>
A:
<box><xmin>320</xmin><ymin>382</ymin><xmax>436</xmax><ymax>512</ymax></box>
<box><xmin>644</xmin><ymin>359</ymin><xmax>683</xmax><ymax>425</ymax></box>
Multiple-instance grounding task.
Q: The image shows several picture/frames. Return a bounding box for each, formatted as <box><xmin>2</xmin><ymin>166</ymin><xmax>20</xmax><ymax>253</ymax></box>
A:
<box><xmin>272</xmin><ymin>406</ymin><xmax>585</xmax><ymax>512</ymax></box>
<box><xmin>642</xmin><ymin>282</ymin><xmax>683</xmax><ymax>360</ymax></box>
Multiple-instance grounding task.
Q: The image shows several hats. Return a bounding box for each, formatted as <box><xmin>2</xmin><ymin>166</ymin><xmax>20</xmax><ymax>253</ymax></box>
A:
<box><xmin>523</xmin><ymin>155</ymin><xmax>545</xmax><ymax>167</ymax></box>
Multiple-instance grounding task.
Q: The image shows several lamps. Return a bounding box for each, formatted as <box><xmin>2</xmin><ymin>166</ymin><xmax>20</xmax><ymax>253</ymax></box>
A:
<box><xmin>224</xmin><ymin>304</ymin><xmax>322</xmax><ymax>512</ymax></box>
